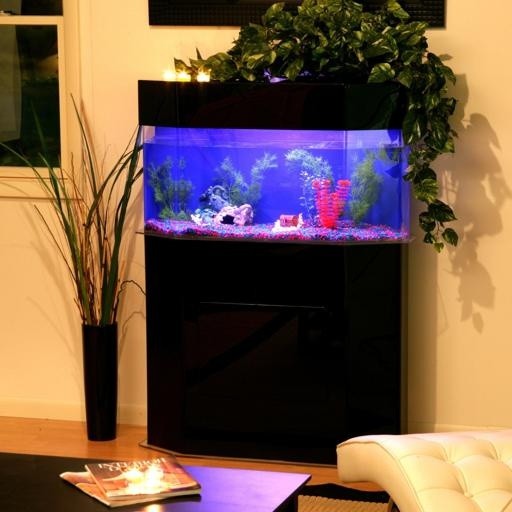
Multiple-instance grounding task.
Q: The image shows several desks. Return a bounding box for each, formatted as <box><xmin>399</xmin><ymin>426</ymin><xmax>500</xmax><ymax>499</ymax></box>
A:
<box><xmin>0</xmin><ymin>451</ymin><xmax>311</xmax><ymax>512</ymax></box>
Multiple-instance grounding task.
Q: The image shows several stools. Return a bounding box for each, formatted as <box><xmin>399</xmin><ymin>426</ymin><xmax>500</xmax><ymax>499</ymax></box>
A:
<box><xmin>335</xmin><ymin>432</ymin><xmax>511</xmax><ymax>512</ymax></box>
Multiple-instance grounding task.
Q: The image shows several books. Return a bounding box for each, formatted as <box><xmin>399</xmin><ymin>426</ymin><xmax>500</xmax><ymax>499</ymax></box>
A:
<box><xmin>86</xmin><ymin>456</ymin><xmax>202</xmax><ymax>500</ymax></box>
<box><xmin>59</xmin><ymin>469</ymin><xmax>169</xmax><ymax>509</ymax></box>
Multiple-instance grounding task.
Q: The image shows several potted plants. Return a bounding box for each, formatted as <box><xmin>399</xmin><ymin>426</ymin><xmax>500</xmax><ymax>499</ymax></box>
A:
<box><xmin>174</xmin><ymin>0</ymin><xmax>460</xmax><ymax>251</ymax></box>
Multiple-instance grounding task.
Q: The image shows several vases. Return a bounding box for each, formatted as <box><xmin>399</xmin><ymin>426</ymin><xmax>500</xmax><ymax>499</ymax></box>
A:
<box><xmin>79</xmin><ymin>322</ymin><xmax>120</xmax><ymax>442</ymax></box>
